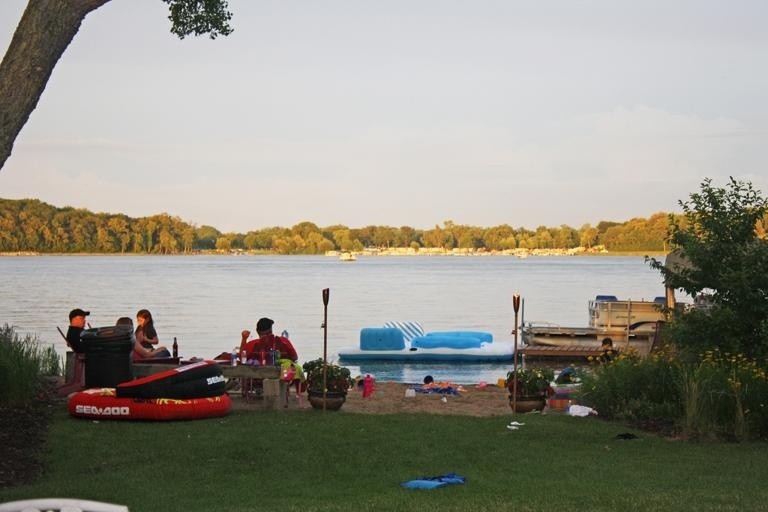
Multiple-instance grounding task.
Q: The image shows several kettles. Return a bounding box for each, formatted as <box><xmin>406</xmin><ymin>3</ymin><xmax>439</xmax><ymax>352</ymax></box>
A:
<box><xmin>364</xmin><ymin>375</ymin><xmax>373</xmax><ymax>398</ymax></box>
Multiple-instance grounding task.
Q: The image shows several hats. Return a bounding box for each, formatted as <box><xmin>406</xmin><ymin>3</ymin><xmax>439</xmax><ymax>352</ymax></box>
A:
<box><xmin>69</xmin><ymin>309</ymin><xmax>90</xmax><ymax>319</ymax></box>
<box><xmin>257</xmin><ymin>318</ymin><xmax>274</xmax><ymax>330</ymax></box>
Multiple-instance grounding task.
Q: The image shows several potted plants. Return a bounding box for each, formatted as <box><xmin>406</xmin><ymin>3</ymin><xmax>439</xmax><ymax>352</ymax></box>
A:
<box><xmin>504</xmin><ymin>366</ymin><xmax>554</xmax><ymax>414</ymax></box>
<box><xmin>302</xmin><ymin>358</ymin><xmax>355</xmax><ymax>410</ymax></box>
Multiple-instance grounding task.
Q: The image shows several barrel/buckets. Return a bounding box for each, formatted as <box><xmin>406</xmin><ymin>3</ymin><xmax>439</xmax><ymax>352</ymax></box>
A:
<box><xmin>80</xmin><ymin>325</ymin><xmax>135</xmax><ymax>387</ymax></box>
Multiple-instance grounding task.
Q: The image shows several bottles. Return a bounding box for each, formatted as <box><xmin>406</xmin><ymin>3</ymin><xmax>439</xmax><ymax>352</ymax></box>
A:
<box><xmin>241</xmin><ymin>351</ymin><xmax>247</xmax><ymax>364</ymax></box>
<box><xmin>267</xmin><ymin>347</ymin><xmax>275</xmax><ymax>366</ymax></box>
<box><xmin>172</xmin><ymin>337</ymin><xmax>178</xmax><ymax>358</ymax></box>
<box><xmin>299</xmin><ymin>392</ymin><xmax>305</xmax><ymax>410</ymax></box>
<box><xmin>231</xmin><ymin>348</ymin><xmax>237</xmax><ymax>367</ymax></box>
<box><xmin>258</xmin><ymin>348</ymin><xmax>265</xmax><ymax>366</ymax></box>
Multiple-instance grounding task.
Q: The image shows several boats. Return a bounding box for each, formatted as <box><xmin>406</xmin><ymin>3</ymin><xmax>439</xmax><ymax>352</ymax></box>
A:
<box><xmin>521</xmin><ymin>294</ymin><xmax>715</xmax><ymax>346</ymax></box>
<box><xmin>338</xmin><ymin>320</ymin><xmax>522</xmax><ymax>361</ymax></box>
<box><xmin>69</xmin><ymin>360</ymin><xmax>232</xmax><ymax>420</ymax></box>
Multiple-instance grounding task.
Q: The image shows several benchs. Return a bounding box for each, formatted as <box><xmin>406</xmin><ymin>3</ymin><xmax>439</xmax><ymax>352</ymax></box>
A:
<box><xmin>64</xmin><ymin>350</ymin><xmax>287</xmax><ymax>412</ymax></box>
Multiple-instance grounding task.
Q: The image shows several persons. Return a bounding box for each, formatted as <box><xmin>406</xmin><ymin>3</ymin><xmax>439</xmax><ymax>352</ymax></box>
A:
<box><xmin>66</xmin><ymin>309</ymin><xmax>90</xmax><ymax>354</ymax></box>
<box><xmin>423</xmin><ymin>375</ymin><xmax>456</xmax><ymax>388</ymax></box>
<box><xmin>600</xmin><ymin>338</ymin><xmax>619</xmax><ymax>363</ymax></box>
<box><xmin>117</xmin><ymin>310</ymin><xmax>170</xmax><ymax>363</ymax></box>
<box><xmin>239</xmin><ymin>318</ymin><xmax>298</xmax><ymax>362</ymax></box>
<box><xmin>663</xmin><ymin>425</ymin><xmax>738</xmax><ymax>444</ymax></box>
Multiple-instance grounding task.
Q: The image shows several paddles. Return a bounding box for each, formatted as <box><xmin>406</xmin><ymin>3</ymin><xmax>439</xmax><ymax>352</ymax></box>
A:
<box><xmin>514</xmin><ymin>293</ymin><xmax>521</xmax><ymax>416</ymax></box>
<box><xmin>322</xmin><ymin>289</ymin><xmax>330</xmax><ymax>410</ymax></box>
